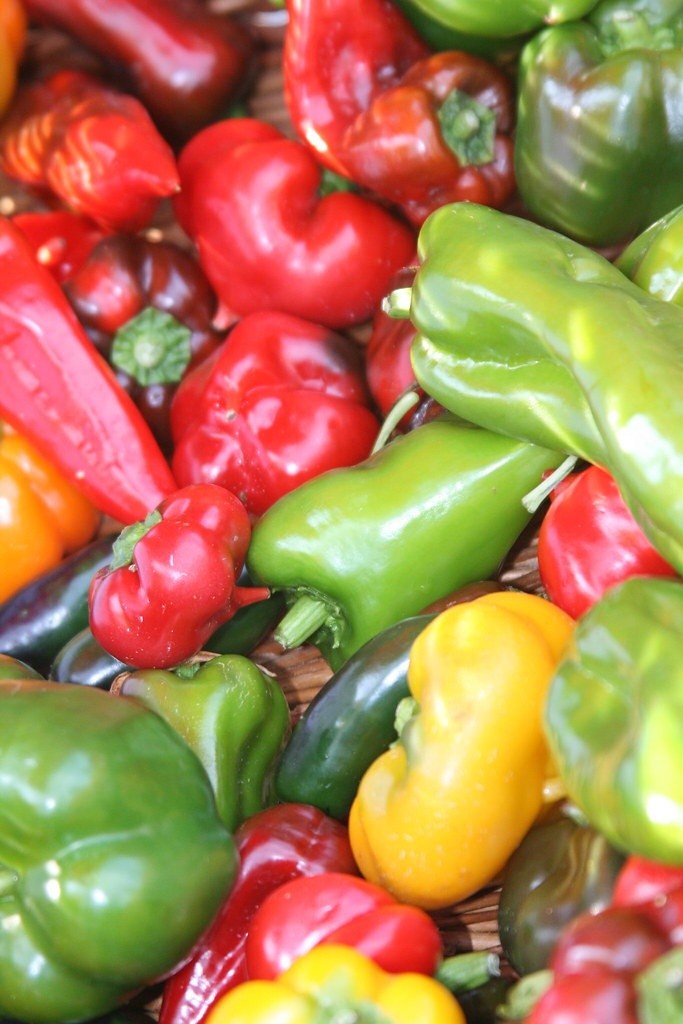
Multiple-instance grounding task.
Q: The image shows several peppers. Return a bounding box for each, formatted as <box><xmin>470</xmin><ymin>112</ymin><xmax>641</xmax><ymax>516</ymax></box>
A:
<box><xmin>0</xmin><ymin>0</ymin><xmax>683</xmax><ymax>1024</ymax></box>
